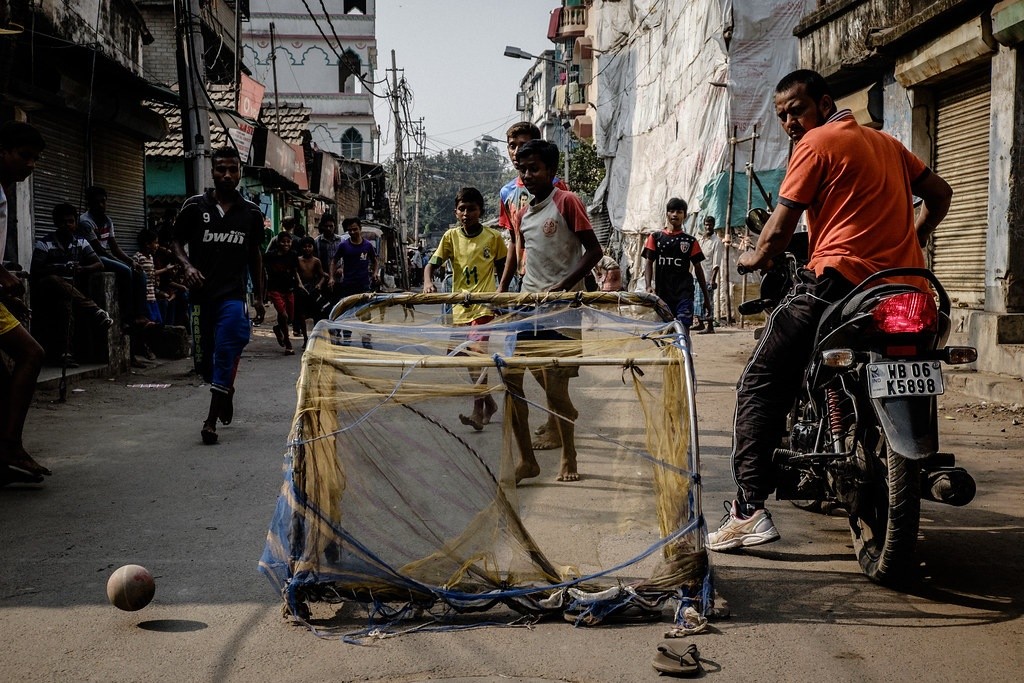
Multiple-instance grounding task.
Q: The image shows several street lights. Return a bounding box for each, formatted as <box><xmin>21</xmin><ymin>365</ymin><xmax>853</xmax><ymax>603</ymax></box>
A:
<box><xmin>503</xmin><ymin>45</ymin><xmax>570</xmax><ymax>193</ymax></box>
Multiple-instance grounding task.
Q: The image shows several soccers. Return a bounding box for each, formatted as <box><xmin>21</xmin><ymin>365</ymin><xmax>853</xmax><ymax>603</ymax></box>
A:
<box><xmin>107</xmin><ymin>565</ymin><xmax>155</xmax><ymax>612</ymax></box>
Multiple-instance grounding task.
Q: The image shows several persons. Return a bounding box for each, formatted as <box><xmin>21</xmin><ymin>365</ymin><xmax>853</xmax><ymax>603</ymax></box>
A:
<box><xmin>702</xmin><ymin>69</ymin><xmax>953</xmax><ymax>551</ymax></box>
<box><xmin>690</xmin><ymin>216</ymin><xmax>723</xmax><ymax>334</ymax></box>
<box><xmin>30</xmin><ymin>186</ymin><xmax>191</xmax><ymax>368</ymax></box>
<box><xmin>582</xmin><ymin>286</ymin><xmax>598</xmax><ymax>331</ymax></box>
<box><xmin>423</xmin><ymin>188</ymin><xmax>508</xmax><ymax>431</ymax></box>
<box><xmin>494</xmin><ymin>122</ymin><xmax>579</xmax><ymax>450</ymax></box>
<box><xmin>501</xmin><ymin>139</ymin><xmax>603</xmax><ymax>486</ymax></box>
<box><xmin>0</xmin><ymin>121</ymin><xmax>52</xmax><ymax>490</ymax></box>
<box><xmin>172</xmin><ymin>146</ymin><xmax>265</xmax><ymax>446</ymax></box>
<box><xmin>407</xmin><ymin>247</ymin><xmax>446</xmax><ymax>288</ymax></box>
<box><xmin>591</xmin><ymin>256</ymin><xmax>622</xmax><ymax>292</ymax></box>
<box><xmin>247</xmin><ymin>212</ymin><xmax>380</xmax><ymax>356</ymax></box>
<box><xmin>640</xmin><ymin>197</ymin><xmax>712</xmax><ymax>333</ymax></box>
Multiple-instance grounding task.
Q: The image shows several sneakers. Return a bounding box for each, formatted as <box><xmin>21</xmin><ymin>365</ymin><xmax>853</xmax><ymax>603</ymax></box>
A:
<box><xmin>704</xmin><ymin>499</ymin><xmax>781</xmax><ymax>551</ymax></box>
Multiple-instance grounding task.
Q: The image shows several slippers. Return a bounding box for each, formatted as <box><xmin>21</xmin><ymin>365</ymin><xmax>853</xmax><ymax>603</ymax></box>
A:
<box><xmin>285</xmin><ymin>348</ymin><xmax>296</xmax><ymax>356</ymax></box>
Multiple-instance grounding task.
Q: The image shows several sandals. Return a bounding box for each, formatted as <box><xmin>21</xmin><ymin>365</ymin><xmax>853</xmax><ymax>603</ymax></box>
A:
<box><xmin>96</xmin><ymin>309</ymin><xmax>114</xmax><ymax>329</ymax></box>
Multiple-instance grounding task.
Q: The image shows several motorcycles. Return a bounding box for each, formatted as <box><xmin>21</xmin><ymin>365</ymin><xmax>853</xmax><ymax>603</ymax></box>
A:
<box><xmin>736</xmin><ymin>197</ymin><xmax>976</xmax><ymax>587</ymax></box>
